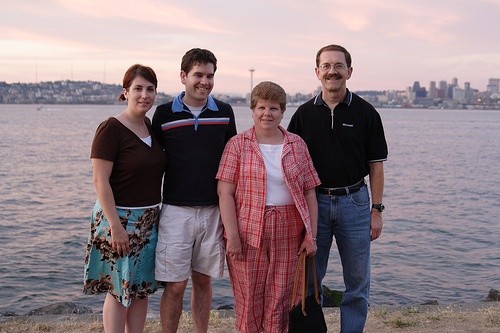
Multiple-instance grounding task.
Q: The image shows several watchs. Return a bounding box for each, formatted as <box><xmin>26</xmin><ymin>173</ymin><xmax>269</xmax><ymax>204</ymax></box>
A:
<box><xmin>372</xmin><ymin>203</ymin><xmax>385</xmax><ymax>212</ymax></box>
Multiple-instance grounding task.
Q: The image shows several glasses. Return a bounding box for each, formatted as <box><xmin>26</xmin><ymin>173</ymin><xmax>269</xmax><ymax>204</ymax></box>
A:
<box><xmin>319</xmin><ymin>64</ymin><xmax>347</xmax><ymax>72</ymax></box>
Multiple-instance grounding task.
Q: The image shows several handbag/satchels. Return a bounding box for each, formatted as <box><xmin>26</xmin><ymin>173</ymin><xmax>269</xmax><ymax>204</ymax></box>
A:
<box><xmin>288</xmin><ymin>294</ymin><xmax>327</xmax><ymax>333</ymax></box>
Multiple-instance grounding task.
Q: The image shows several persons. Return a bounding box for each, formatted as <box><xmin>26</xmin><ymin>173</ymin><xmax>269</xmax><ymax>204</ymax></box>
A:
<box><xmin>216</xmin><ymin>81</ymin><xmax>321</xmax><ymax>333</ymax></box>
<box><xmin>83</xmin><ymin>64</ymin><xmax>164</xmax><ymax>333</ymax></box>
<box><xmin>287</xmin><ymin>45</ymin><xmax>388</xmax><ymax>333</ymax></box>
<box><xmin>152</xmin><ymin>48</ymin><xmax>237</xmax><ymax>333</ymax></box>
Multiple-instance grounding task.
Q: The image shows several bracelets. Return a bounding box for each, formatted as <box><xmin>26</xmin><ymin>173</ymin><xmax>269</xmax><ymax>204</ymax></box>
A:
<box><xmin>313</xmin><ymin>238</ymin><xmax>316</xmax><ymax>240</ymax></box>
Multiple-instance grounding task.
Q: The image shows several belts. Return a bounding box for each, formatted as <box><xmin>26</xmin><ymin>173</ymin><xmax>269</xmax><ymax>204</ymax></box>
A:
<box><xmin>318</xmin><ymin>179</ymin><xmax>364</xmax><ymax>196</ymax></box>
